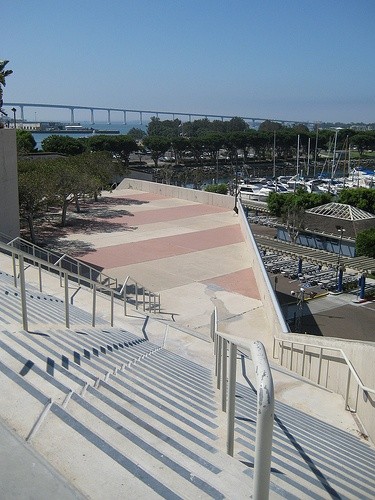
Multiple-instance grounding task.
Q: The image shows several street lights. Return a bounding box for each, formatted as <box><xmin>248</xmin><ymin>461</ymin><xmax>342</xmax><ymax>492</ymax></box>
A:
<box><xmin>291</xmin><ymin>287</ymin><xmax>303</xmax><ymax>334</ymax></box>
<box><xmin>337</xmin><ymin>226</ymin><xmax>346</xmax><ymax>265</ymax></box>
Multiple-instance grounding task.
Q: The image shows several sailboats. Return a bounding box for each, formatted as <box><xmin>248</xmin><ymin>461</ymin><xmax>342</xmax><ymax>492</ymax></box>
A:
<box><xmin>231</xmin><ymin>123</ymin><xmax>375</xmax><ymax>203</ymax></box>
